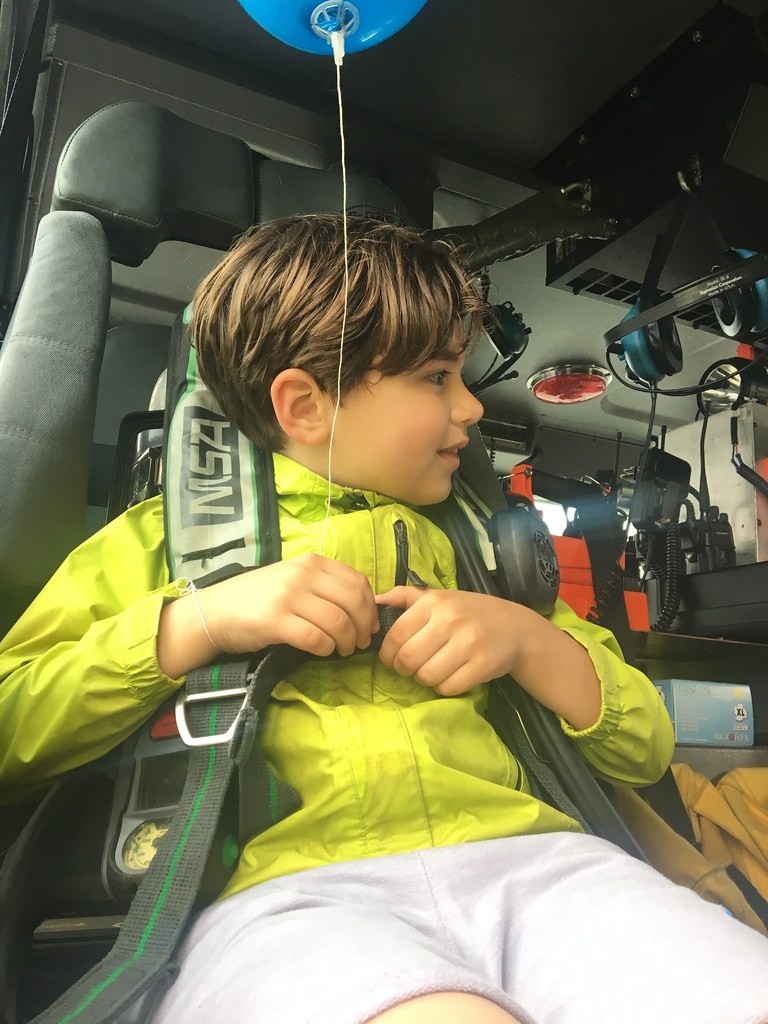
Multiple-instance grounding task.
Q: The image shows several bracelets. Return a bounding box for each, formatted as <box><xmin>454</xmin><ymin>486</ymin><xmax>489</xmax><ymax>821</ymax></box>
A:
<box><xmin>174</xmin><ymin>577</ymin><xmax>218</xmax><ymax>649</ymax></box>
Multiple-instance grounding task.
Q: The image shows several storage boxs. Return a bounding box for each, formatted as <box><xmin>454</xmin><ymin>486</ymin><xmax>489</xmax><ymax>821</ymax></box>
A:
<box><xmin>636</xmin><ymin>678</ymin><xmax>755</xmax><ymax>752</ymax></box>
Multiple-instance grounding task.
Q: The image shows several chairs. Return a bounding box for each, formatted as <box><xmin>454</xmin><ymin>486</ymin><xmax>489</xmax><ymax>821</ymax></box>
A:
<box><xmin>0</xmin><ymin>97</ymin><xmax>651</xmax><ymax>1024</ymax></box>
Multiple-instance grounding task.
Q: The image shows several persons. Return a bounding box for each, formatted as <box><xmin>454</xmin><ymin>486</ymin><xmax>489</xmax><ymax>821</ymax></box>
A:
<box><xmin>0</xmin><ymin>213</ymin><xmax>768</xmax><ymax>1024</ymax></box>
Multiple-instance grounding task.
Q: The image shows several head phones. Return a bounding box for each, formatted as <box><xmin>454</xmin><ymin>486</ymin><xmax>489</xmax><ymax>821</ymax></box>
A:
<box><xmin>470</xmin><ymin>271</ymin><xmax>532</xmax><ymax>395</ymax></box>
<box><xmin>620</xmin><ymin>183</ymin><xmax>767</xmax><ymax>384</ymax></box>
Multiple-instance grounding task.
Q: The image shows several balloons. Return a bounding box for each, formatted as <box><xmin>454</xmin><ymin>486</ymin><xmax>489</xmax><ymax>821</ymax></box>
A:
<box><xmin>236</xmin><ymin>0</ymin><xmax>428</xmax><ymax>56</ymax></box>
